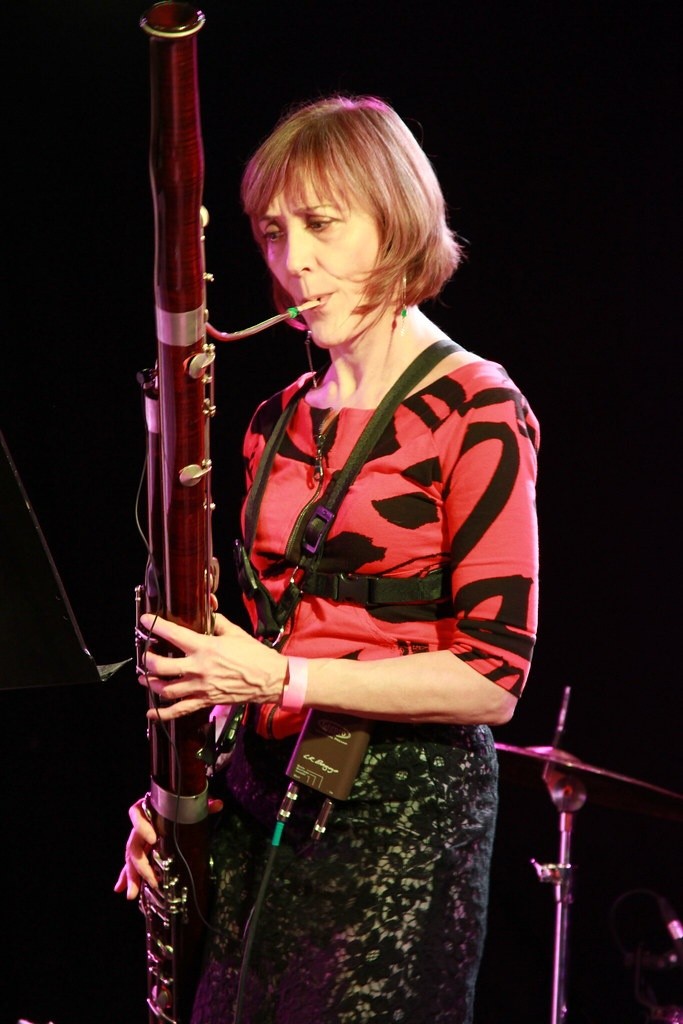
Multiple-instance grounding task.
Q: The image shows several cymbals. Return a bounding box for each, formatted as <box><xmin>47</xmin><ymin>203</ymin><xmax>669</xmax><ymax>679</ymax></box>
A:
<box><xmin>493</xmin><ymin>743</ymin><xmax>683</xmax><ymax>799</ymax></box>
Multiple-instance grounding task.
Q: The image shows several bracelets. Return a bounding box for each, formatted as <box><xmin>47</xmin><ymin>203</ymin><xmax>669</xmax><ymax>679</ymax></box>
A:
<box><xmin>281</xmin><ymin>655</ymin><xmax>307</xmax><ymax>714</ymax></box>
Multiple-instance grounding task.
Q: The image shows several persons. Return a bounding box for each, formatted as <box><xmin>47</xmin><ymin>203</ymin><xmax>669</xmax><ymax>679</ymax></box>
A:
<box><xmin>112</xmin><ymin>97</ymin><xmax>541</xmax><ymax>1024</ymax></box>
